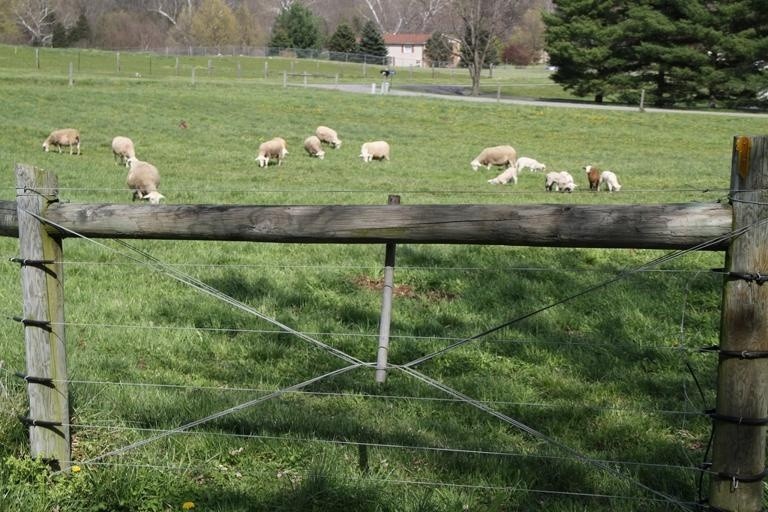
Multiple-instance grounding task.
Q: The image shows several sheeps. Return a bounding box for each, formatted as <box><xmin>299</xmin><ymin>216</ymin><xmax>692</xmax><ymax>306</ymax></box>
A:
<box><xmin>255</xmin><ymin>137</ymin><xmax>288</xmax><ymax>167</ymax></box>
<box><xmin>359</xmin><ymin>141</ymin><xmax>390</xmax><ymax>163</ymax></box>
<box><xmin>42</xmin><ymin>128</ymin><xmax>80</xmax><ymax>156</ymax></box>
<box><xmin>305</xmin><ymin>136</ymin><xmax>325</xmax><ymax>159</ymax></box>
<box><xmin>316</xmin><ymin>125</ymin><xmax>342</xmax><ymax>150</ymax></box>
<box><xmin>470</xmin><ymin>145</ymin><xmax>623</xmax><ymax>194</ymax></box>
<box><xmin>112</xmin><ymin>136</ymin><xmax>138</xmax><ymax>168</ymax></box>
<box><xmin>127</xmin><ymin>161</ymin><xmax>165</xmax><ymax>205</ymax></box>
